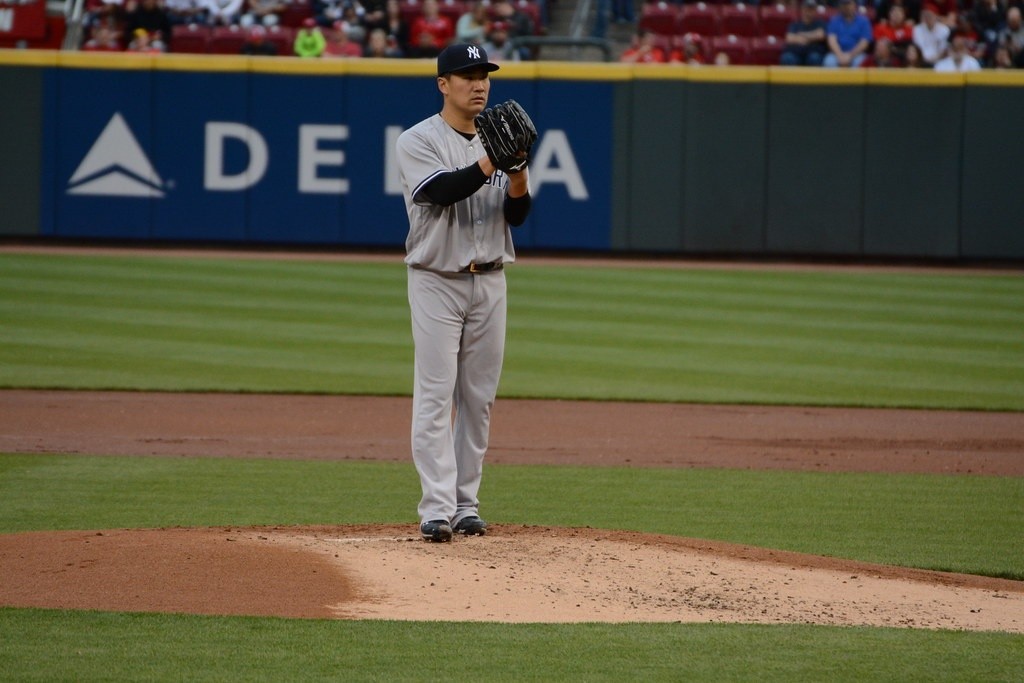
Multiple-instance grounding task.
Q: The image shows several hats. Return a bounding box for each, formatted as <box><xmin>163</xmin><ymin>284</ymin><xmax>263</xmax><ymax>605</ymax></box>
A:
<box><xmin>437</xmin><ymin>43</ymin><xmax>499</xmax><ymax>77</ymax></box>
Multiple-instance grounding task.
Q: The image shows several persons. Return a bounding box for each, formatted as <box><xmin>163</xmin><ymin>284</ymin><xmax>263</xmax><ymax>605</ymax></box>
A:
<box><xmin>0</xmin><ymin>0</ymin><xmax>1024</xmax><ymax>71</ymax></box>
<box><xmin>396</xmin><ymin>43</ymin><xmax>538</xmax><ymax>543</ymax></box>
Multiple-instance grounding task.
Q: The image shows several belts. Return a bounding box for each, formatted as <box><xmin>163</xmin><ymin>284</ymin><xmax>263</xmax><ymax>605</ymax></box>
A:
<box><xmin>460</xmin><ymin>262</ymin><xmax>503</xmax><ymax>273</ymax></box>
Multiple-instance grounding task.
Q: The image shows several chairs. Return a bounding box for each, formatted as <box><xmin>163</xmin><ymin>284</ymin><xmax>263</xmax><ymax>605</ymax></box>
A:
<box><xmin>170</xmin><ymin>0</ymin><xmax>540</xmax><ymax>56</ymax></box>
<box><xmin>637</xmin><ymin>0</ymin><xmax>837</xmax><ymax>66</ymax></box>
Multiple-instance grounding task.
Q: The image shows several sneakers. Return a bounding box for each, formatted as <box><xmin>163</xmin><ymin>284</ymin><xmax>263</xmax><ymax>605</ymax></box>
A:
<box><xmin>421</xmin><ymin>520</ymin><xmax>453</xmax><ymax>540</ymax></box>
<box><xmin>454</xmin><ymin>517</ymin><xmax>487</xmax><ymax>534</ymax></box>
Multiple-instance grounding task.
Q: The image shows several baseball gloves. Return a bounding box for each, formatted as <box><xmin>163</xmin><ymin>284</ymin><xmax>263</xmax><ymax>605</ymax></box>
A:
<box><xmin>473</xmin><ymin>98</ymin><xmax>539</xmax><ymax>175</ymax></box>
